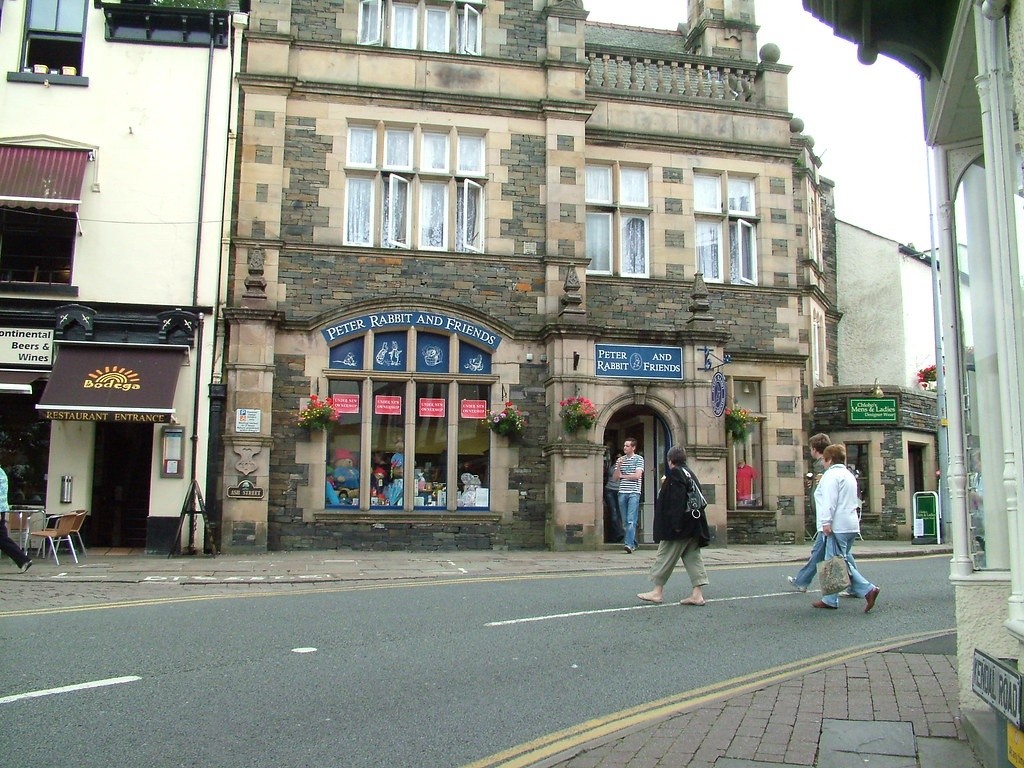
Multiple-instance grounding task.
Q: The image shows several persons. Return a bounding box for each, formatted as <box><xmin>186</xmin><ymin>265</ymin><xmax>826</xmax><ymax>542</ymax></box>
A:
<box><xmin>970</xmin><ymin>449</ymin><xmax>985</xmax><ymax>552</ymax></box>
<box><xmin>612</xmin><ymin>438</ymin><xmax>645</xmax><ymax>553</ymax></box>
<box><xmin>604</xmin><ymin>449</ymin><xmax>624</xmax><ymax>543</ymax></box>
<box><xmin>637</xmin><ymin>444</ymin><xmax>710</xmax><ymax>606</ymax></box>
<box><xmin>0</xmin><ymin>467</ymin><xmax>33</xmax><ymax>573</ymax></box>
<box><xmin>788</xmin><ymin>433</ymin><xmax>857</xmax><ymax>598</ymax></box>
<box><xmin>736</xmin><ymin>460</ymin><xmax>757</xmax><ymax>500</ymax></box>
<box><xmin>812</xmin><ymin>444</ymin><xmax>881</xmax><ymax>612</ymax></box>
<box><xmin>371</xmin><ymin>450</ymin><xmax>391</xmax><ymax>492</ymax></box>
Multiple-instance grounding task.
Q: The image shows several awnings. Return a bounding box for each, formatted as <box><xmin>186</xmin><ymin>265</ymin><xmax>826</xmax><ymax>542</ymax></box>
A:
<box><xmin>0</xmin><ymin>369</ymin><xmax>48</xmax><ymax>394</ymax></box>
<box><xmin>0</xmin><ymin>143</ymin><xmax>93</xmax><ymax>235</ymax></box>
<box><xmin>35</xmin><ymin>339</ymin><xmax>190</xmax><ymax>424</ymax></box>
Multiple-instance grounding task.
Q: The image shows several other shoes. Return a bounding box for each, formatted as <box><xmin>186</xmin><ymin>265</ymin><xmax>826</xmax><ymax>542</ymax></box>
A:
<box><xmin>787</xmin><ymin>574</ymin><xmax>808</xmax><ymax>593</ymax></box>
<box><xmin>812</xmin><ymin>600</ymin><xmax>834</xmax><ymax>609</ymax></box>
<box><xmin>637</xmin><ymin>592</ymin><xmax>662</xmax><ymax>603</ymax></box>
<box><xmin>624</xmin><ymin>545</ymin><xmax>633</xmax><ymax>553</ymax></box>
<box><xmin>863</xmin><ymin>586</ymin><xmax>881</xmax><ymax>613</ymax></box>
<box><xmin>837</xmin><ymin>589</ymin><xmax>853</xmax><ymax>596</ymax></box>
<box><xmin>679</xmin><ymin>597</ymin><xmax>705</xmax><ymax>606</ymax></box>
<box><xmin>18</xmin><ymin>559</ymin><xmax>33</xmax><ymax>574</ymax></box>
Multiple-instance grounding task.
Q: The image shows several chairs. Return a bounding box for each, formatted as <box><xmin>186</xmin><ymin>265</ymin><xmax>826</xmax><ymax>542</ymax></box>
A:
<box><xmin>6</xmin><ymin>507</ymin><xmax>30</xmax><ymax>553</ymax></box>
<box><xmin>51</xmin><ymin>509</ymin><xmax>89</xmax><ymax>558</ymax></box>
<box><xmin>856</xmin><ymin>499</ymin><xmax>864</xmax><ymax>541</ymax></box>
<box><xmin>30</xmin><ymin>513</ymin><xmax>79</xmax><ymax>566</ymax></box>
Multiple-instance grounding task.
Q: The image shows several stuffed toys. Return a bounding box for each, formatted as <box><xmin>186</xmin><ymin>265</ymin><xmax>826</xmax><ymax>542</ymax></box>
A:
<box><xmin>327</xmin><ymin>436</ymin><xmax>404</xmax><ymax>497</ymax></box>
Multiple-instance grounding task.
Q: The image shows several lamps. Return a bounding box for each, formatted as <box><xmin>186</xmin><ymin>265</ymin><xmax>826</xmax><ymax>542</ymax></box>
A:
<box><xmin>871</xmin><ymin>378</ymin><xmax>885</xmax><ymax>399</ymax></box>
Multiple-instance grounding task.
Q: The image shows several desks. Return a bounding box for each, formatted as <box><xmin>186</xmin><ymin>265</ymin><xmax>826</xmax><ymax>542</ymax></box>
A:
<box><xmin>6</xmin><ymin>509</ymin><xmax>40</xmax><ymax>550</ymax></box>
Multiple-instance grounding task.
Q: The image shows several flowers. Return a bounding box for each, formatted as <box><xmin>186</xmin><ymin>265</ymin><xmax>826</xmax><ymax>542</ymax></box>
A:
<box><xmin>558</xmin><ymin>397</ymin><xmax>598</xmax><ymax>433</ymax></box>
<box><xmin>289</xmin><ymin>392</ymin><xmax>342</xmax><ymax>434</ymax></box>
<box><xmin>917</xmin><ymin>364</ymin><xmax>937</xmax><ymax>385</ymax></box>
<box><xmin>724</xmin><ymin>406</ymin><xmax>763</xmax><ymax>444</ymax></box>
<box><xmin>480</xmin><ymin>402</ymin><xmax>531</xmax><ymax>437</ymax></box>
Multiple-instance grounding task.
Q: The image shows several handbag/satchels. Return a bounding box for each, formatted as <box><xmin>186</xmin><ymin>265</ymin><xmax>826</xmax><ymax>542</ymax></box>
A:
<box><xmin>816</xmin><ymin>533</ymin><xmax>852</xmax><ymax>596</ymax></box>
<box><xmin>678</xmin><ymin>466</ymin><xmax>708</xmax><ymax>514</ymax></box>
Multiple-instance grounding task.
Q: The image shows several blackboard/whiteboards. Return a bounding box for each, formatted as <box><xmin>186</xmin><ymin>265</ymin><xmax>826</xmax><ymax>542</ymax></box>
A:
<box><xmin>168</xmin><ymin>480</ymin><xmax>219</xmax><ymax>559</ymax></box>
